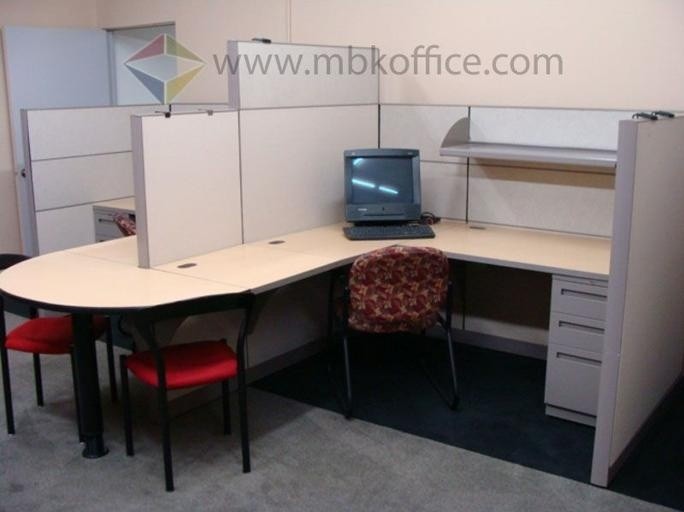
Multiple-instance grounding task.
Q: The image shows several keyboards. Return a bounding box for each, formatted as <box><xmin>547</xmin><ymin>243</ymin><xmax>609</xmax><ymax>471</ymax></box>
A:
<box><xmin>341</xmin><ymin>225</ymin><xmax>435</xmax><ymax>241</ymax></box>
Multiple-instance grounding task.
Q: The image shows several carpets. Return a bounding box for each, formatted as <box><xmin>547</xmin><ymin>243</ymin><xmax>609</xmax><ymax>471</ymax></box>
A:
<box><xmin>2</xmin><ymin>309</ymin><xmax>675</xmax><ymax>512</ymax></box>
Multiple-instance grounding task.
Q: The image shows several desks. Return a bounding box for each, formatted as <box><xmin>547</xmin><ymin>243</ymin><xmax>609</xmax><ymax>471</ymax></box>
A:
<box><xmin>1</xmin><ymin>193</ymin><xmax>612</xmax><ymax>434</ymax></box>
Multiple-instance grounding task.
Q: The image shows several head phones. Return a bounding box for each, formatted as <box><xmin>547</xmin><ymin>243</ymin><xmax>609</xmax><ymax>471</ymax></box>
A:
<box><xmin>419</xmin><ymin>211</ymin><xmax>436</xmax><ymax>225</ymax></box>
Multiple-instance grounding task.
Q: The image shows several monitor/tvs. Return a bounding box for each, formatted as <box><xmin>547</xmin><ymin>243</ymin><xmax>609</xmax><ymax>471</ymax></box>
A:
<box><xmin>344</xmin><ymin>148</ymin><xmax>422</xmax><ymax>224</ymax></box>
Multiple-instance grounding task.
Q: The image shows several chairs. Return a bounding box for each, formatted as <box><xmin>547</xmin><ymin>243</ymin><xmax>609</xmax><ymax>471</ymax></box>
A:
<box><xmin>121</xmin><ymin>292</ymin><xmax>263</xmax><ymax>492</ymax></box>
